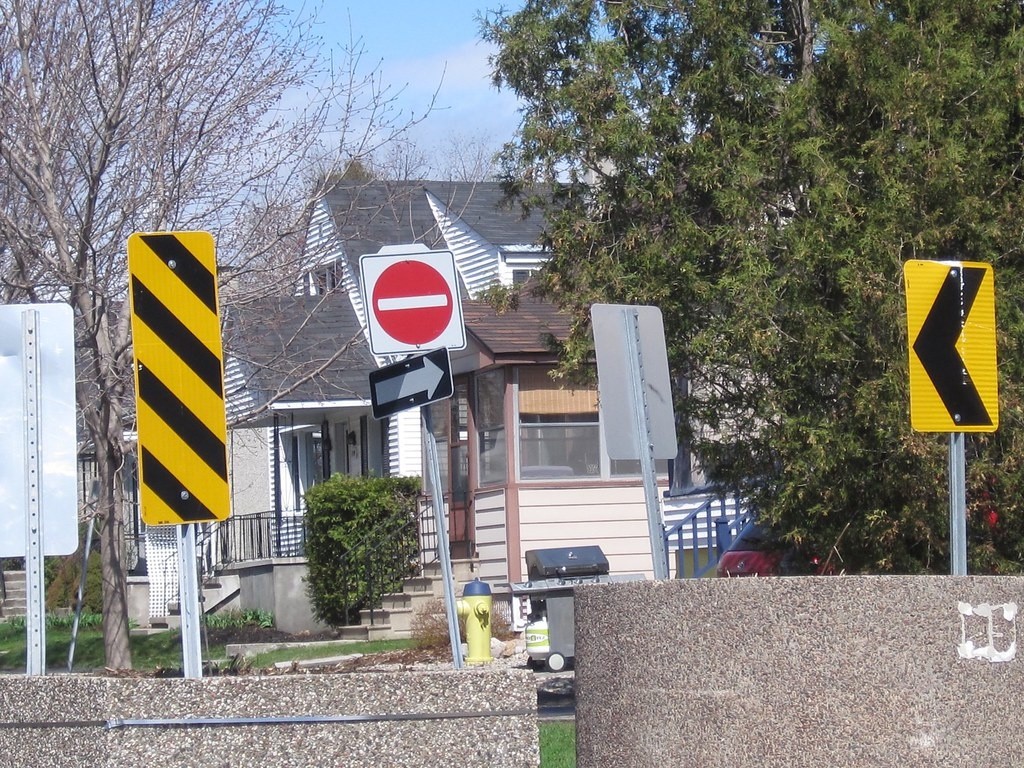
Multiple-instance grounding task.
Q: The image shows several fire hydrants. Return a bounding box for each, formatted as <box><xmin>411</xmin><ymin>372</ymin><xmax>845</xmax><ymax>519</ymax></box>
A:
<box><xmin>456</xmin><ymin>578</ymin><xmax>495</xmax><ymax>663</ymax></box>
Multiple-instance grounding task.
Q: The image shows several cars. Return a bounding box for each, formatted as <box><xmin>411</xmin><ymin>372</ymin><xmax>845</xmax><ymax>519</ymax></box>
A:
<box><xmin>715</xmin><ymin>517</ymin><xmax>834</xmax><ymax>578</ymax></box>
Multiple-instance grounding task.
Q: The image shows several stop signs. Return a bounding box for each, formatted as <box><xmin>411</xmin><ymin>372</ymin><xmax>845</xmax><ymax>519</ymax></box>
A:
<box><xmin>360</xmin><ymin>251</ymin><xmax>467</xmax><ymax>356</ymax></box>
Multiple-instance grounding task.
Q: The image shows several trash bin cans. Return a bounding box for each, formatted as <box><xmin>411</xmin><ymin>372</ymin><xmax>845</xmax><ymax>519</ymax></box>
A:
<box><xmin>523</xmin><ymin>545</ymin><xmax>611</xmax><ymax>673</ymax></box>
